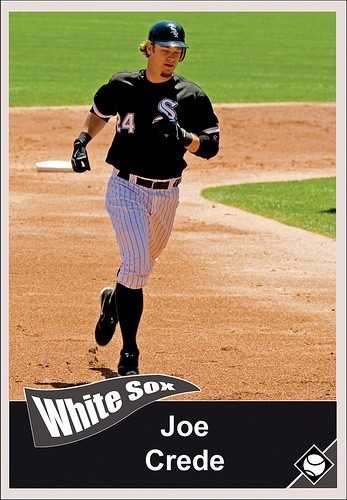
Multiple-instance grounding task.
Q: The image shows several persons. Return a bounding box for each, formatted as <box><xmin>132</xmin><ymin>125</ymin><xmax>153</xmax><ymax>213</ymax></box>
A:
<box><xmin>71</xmin><ymin>20</ymin><xmax>220</xmax><ymax>378</ymax></box>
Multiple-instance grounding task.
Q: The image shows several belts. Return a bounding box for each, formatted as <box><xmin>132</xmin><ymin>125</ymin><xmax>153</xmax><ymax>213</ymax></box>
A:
<box><xmin>117</xmin><ymin>170</ymin><xmax>182</xmax><ymax>189</ymax></box>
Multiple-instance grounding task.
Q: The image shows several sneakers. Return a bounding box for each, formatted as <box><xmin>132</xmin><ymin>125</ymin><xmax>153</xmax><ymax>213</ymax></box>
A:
<box><xmin>117</xmin><ymin>350</ymin><xmax>139</xmax><ymax>375</ymax></box>
<box><xmin>95</xmin><ymin>288</ymin><xmax>118</xmax><ymax>345</ymax></box>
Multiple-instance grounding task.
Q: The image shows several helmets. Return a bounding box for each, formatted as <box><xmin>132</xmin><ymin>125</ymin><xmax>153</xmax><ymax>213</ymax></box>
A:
<box><xmin>147</xmin><ymin>21</ymin><xmax>189</xmax><ymax>63</ymax></box>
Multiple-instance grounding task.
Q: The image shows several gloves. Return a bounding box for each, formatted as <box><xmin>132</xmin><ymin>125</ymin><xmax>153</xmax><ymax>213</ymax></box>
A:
<box><xmin>71</xmin><ymin>139</ymin><xmax>90</xmax><ymax>173</ymax></box>
<box><xmin>151</xmin><ymin>113</ymin><xmax>192</xmax><ymax>147</ymax></box>
